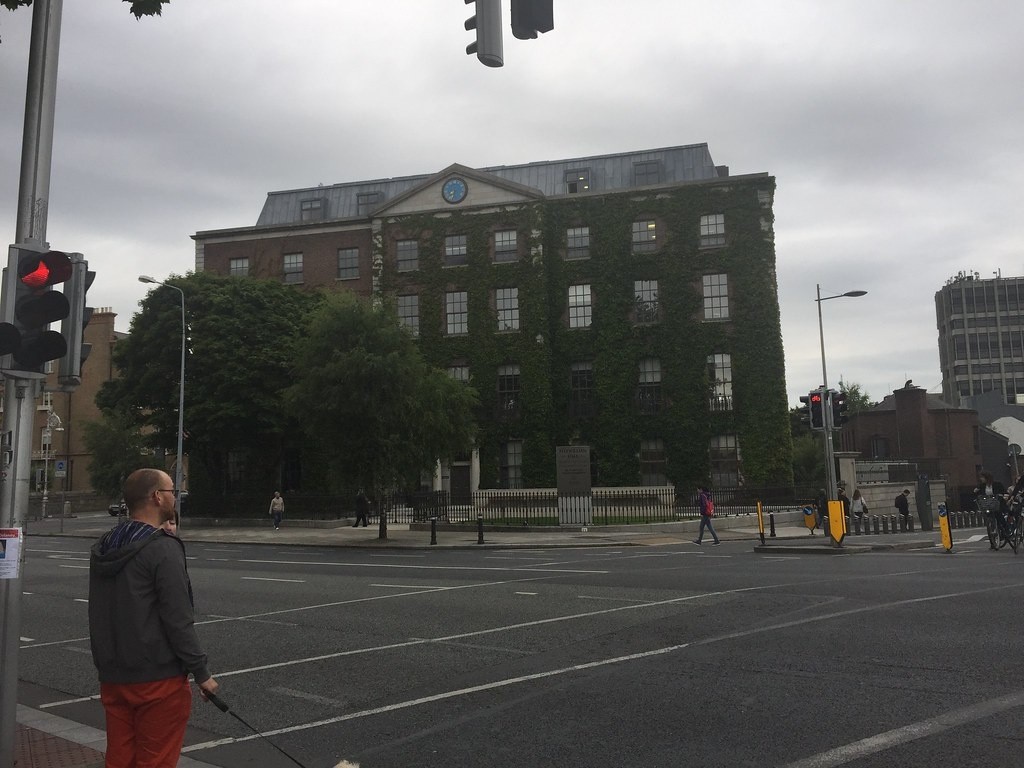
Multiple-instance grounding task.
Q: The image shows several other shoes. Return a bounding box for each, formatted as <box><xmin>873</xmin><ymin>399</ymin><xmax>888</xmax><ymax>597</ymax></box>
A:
<box><xmin>1007</xmin><ymin>529</ymin><xmax>1013</xmax><ymax>536</ymax></box>
<box><xmin>991</xmin><ymin>541</ymin><xmax>995</xmax><ymax>549</ymax></box>
<box><xmin>274</xmin><ymin>523</ymin><xmax>279</xmax><ymax>530</ymax></box>
<box><xmin>692</xmin><ymin>540</ymin><xmax>701</xmax><ymax>546</ymax></box>
<box><xmin>352</xmin><ymin>524</ymin><xmax>357</xmax><ymax>527</ymax></box>
<box><xmin>712</xmin><ymin>543</ymin><xmax>721</xmax><ymax>546</ymax></box>
<box><xmin>816</xmin><ymin>523</ymin><xmax>820</xmax><ymax>530</ymax></box>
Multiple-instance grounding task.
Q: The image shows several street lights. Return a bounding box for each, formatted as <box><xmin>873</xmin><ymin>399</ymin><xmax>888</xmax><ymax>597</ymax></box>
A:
<box><xmin>138</xmin><ymin>274</ymin><xmax>186</xmax><ymax>536</ymax></box>
<box><xmin>815</xmin><ymin>284</ymin><xmax>868</xmax><ymax>500</ymax></box>
<box><xmin>41</xmin><ymin>409</ymin><xmax>64</xmax><ymax>518</ymax></box>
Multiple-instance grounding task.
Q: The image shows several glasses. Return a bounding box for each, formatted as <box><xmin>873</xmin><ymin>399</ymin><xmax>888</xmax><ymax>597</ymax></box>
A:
<box><xmin>152</xmin><ymin>489</ymin><xmax>179</xmax><ymax>499</ymax></box>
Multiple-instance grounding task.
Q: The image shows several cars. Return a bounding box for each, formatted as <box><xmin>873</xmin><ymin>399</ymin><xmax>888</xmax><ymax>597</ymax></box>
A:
<box><xmin>108</xmin><ymin>497</ymin><xmax>129</xmax><ymax>516</ymax></box>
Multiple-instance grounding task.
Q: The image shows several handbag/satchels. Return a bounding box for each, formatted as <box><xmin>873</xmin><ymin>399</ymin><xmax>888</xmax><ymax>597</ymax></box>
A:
<box><xmin>863</xmin><ymin>505</ymin><xmax>868</xmax><ymax>514</ymax></box>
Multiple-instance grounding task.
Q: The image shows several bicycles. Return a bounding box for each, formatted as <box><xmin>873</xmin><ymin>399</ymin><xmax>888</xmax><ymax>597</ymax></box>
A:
<box><xmin>974</xmin><ymin>490</ymin><xmax>1024</xmax><ymax>555</ymax></box>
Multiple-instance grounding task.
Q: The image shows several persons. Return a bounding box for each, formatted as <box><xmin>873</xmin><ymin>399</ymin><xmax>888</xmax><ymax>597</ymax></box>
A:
<box><xmin>352</xmin><ymin>487</ymin><xmax>368</xmax><ymax>527</ymax></box>
<box><xmin>850</xmin><ymin>489</ymin><xmax>868</xmax><ymax>528</ymax></box>
<box><xmin>692</xmin><ymin>486</ymin><xmax>721</xmax><ymax>546</ymax></box>
<box><xmin>88</xmin><ymin>468</ymin><xmax>219</xmax><ymax>768</ymax></box>
<box><xmin>971</xmin><ymin>470</ymin><xmax>1006</xmax><ymax>500</ymax></box>
<box><xmin>895</xmin><ymin>489</ymin><xmax>912</xmax><ymax>531</ymax></box>
<box><xmin>835</xmin><ymin>488</ymin><xmax>851</xmax><ymax>518</ymax></box>
<box><xmin>1003</xmin><ymin>476</ymin><xmax>1024</xmax><ymax>518</ymax></box>
<box><xmin>269</xmin><ymin>491</ymin><xmax>285</xmax><ymax>530</ymax></box>
<box><xmin>814</xmin><ymin>488</ymin><xmax>829</xmax><ymax>528</ymax></box>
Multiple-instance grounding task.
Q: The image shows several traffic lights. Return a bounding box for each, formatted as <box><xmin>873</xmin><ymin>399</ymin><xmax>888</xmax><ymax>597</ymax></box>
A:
<box><xmin>463</xmin><ymin>0</ymin><xmax>504</xmax><ymax>67</ymax></box>
<box><xmin>800</xmin><ymin>394</ymin><xmax>812</xmax><ymax>429</ymax></box>
<box><xmin>59</xmin><ymin>252</ymin><xmax>97</xmax><ymax>386</ymax></box>
<box><xmin>0</xmin><ymin>241</ymin><xmax>73</xmax><ymax>379</ymax></box>
<box><xmin>807</xmin><ymin>388</ymin><xmax>827</xmax><ymax>430</ymax></box>
<box><xmin>829</xmin><ymin>389</ymin><xmax>849</xmax><ymax>430</ymax></box>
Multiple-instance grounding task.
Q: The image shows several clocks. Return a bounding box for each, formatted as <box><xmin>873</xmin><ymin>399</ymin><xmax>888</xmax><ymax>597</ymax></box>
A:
<box><xmin>442</xmin><ymin>177</ymin><xmax>468</xmax><ymax>204</ymax></box>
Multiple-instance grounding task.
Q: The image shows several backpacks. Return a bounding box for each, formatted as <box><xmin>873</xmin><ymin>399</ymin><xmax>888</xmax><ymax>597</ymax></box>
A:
<box><xmin>895</xmin><ymin>495</ymin><xmax>904</xmax><ymax>508</ymax></box>
<box><xmin>702</xmin><ymin>494</ymin><xmax>713</xmax><ymax>517</ymax></box>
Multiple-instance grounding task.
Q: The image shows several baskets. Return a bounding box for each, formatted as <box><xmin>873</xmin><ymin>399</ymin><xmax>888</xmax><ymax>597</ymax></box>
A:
<box><xmin>976</xmin><ymin>499</ymin><xmax>1000</xmax><ymax>512</ymax></box>
